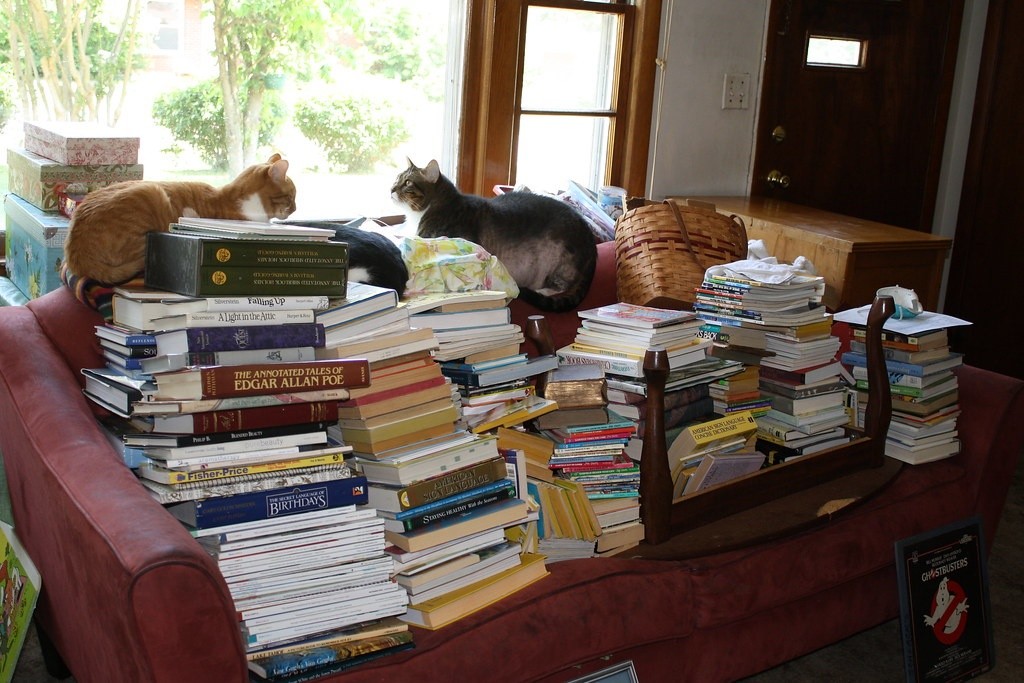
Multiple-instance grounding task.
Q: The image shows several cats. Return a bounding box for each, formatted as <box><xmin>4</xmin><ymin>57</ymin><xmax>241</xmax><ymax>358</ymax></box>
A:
<box><xmin>270</xmin><ymin>218</ymin><xmax>409</xmax><ymax>304</ymax></box>
<box><xmin>389</xmin><ymin>155</ymin><xmax>599</xmax><ymax>313</ymax></box>
<box><xmin>64</xmin><ymin>151</ymin><xmax>297</xmax><ymax>287</ymax></box>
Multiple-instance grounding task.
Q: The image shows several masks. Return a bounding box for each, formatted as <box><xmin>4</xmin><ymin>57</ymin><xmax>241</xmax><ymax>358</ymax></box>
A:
<box><xmin>876</xmin><ymin>284</ymin><xmax>924</xmax><ymax>322</ymax></box>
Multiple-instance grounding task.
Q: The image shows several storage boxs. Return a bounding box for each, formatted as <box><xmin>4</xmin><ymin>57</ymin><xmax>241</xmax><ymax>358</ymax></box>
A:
<box><xmin>145</xmin><ymin>231</ymin><xmax>349</xmax><ymax>299</ymax></box>
<box><xmin>4</xmin><ymin>193</ymin><xmax>72</xmax><ymax>300</ymax></box>
<box><xmin>6</xmin><ymin>146</ymin><xmax>143</xmax><ymax>212</ymax></box>
<box><xmin>58</xmin><ymin>191</ymin><xmax>85</xmax><ymax>217</ymax></box>
<box><xmin>24</xmin><ymin>121</ymin><xmax>141</xmax><ymax>167</ymax></box>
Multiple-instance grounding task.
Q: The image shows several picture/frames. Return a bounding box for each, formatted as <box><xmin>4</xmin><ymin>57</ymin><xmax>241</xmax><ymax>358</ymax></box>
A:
<box><xmin>892</xmin><ymin>514</ymin><xmax>996</xmax><ymax>683</ymax></box>
<box><xmin>566</xmin><ymin>658</ymin><xmax>639</xmax><ymax>683</ymax></box>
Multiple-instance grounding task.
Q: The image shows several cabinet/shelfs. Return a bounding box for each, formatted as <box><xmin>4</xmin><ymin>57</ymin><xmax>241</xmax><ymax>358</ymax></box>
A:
<box><xmin>665</xmin><ymin>195</ymin><xmax>953</xmax><ymax>316</ymax></box>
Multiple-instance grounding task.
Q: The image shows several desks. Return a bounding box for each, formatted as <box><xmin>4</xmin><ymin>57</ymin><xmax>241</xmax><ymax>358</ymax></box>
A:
<box><xmin>525</xmin><ymin>296</ymin><xmax>904</xmax><ymax>561</ymax></box>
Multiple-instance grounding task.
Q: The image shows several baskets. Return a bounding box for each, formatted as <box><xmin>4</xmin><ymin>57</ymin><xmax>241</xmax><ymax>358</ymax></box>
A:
<box><xmin>613</xmin><ymin>198</ymin><xmax>748</xmax><ymax>311</ymax></box>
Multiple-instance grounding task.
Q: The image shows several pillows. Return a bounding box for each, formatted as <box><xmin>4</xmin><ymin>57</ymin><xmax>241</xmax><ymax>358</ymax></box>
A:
<box><xmin>389</xmin><ymin>234</ymin><xmax>521</xmax><ymax>299</ymax></box>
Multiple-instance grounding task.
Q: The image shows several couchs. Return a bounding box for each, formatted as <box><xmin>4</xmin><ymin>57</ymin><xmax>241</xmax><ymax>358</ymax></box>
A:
<box><xmin>0</xmin><ymin>239</ymin><xmax>1024</xmax><ymax>683</ymax></box>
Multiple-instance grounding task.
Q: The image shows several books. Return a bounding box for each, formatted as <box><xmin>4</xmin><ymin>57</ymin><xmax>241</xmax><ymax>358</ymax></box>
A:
<box><xmin>75</xmin><ymin>212</ymin><xmax>995</xmax><ymax>683</ymax></box>
<box><xmin>0</xmin><ymin>521</ymin><xmax>42</xmax><ymax>683</ymax></box>
